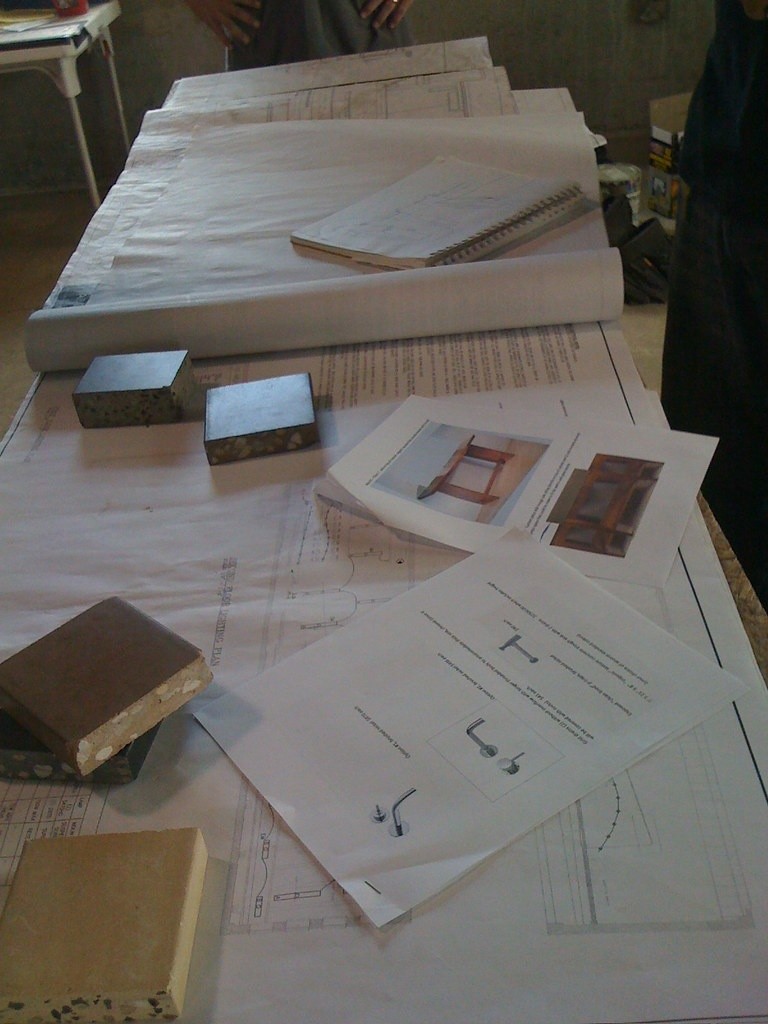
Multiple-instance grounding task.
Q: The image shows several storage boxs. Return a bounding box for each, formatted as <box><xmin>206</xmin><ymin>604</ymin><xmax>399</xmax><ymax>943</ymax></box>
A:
<box><xmin>643</xmin><ymin>90</ymin><xmax>699</xmax><ymax>232</ymax></box>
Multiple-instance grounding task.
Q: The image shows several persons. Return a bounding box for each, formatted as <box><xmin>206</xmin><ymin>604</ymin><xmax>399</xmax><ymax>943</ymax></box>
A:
<box><xmin>181</xmin><ymin>0</ymin><xmax>415</xmax><ymax>71</ymax></box>
<box><xmin>659</xmin><ymin>1</ymin><xmax>768</xmax><ymax>612</ymax></box>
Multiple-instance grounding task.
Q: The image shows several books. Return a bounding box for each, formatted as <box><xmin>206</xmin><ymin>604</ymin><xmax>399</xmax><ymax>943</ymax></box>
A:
<box><xmin>291</xmin><ymin>154</ymin><xmax>588</xmax><ymax>272</ymax></box>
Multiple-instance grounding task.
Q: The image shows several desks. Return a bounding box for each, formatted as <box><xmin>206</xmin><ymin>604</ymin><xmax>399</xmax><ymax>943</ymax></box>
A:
<box><xmin>1</xmin><ymin>86</ymin><xmax>767</xmax><ymax>1024</ymax></box>
<box><xmin>0</xmin><ymin>0</ymin><xmax>128</xmax><ymax>206</ymax></box>
